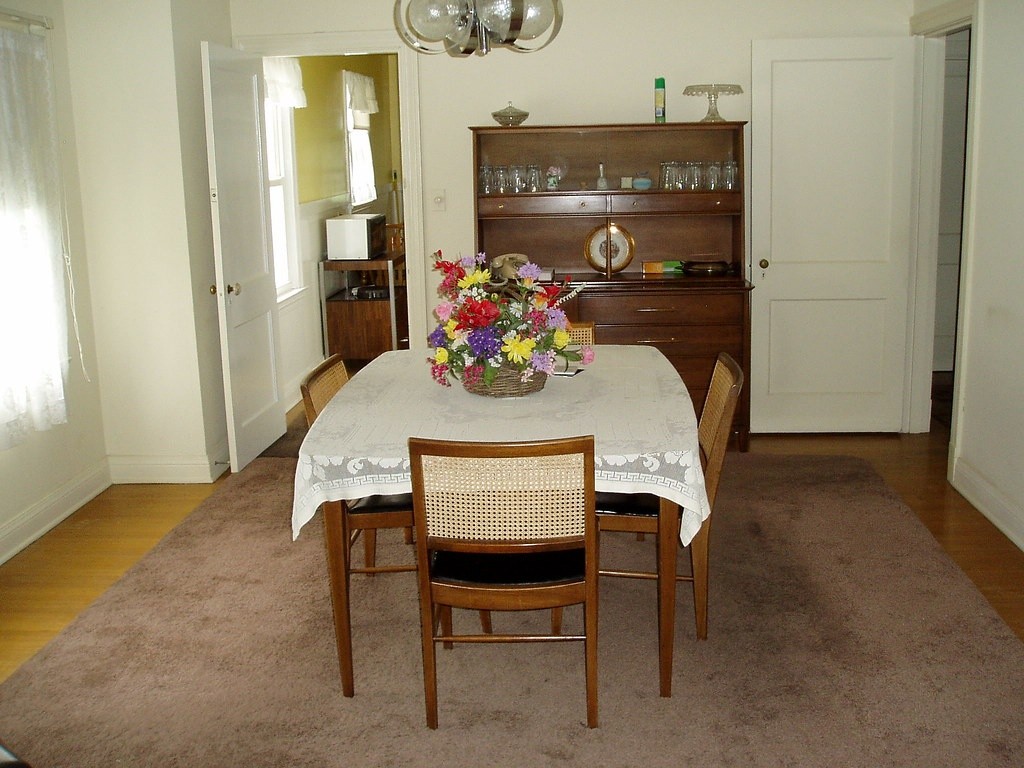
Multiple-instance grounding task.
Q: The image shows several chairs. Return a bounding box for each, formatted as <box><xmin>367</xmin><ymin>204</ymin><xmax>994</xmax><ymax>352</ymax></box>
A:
<box><xmin>549</xmin><ymin>347</ymin><xmax>746</xmax><ymax>645</ymax></box>
<box><xmin>298</xmin><ymin>351</ymin><xmax>414</xmax><ymax>579</ymax></box>
<box><xmin>405</xmin><ymin>435</ymin><xmax>602</xmax><ymax>734</ymax></box>
<box><xmin>566</xmin><ymin>320</ymin><xmax>596</xmax><ymax>346</ymax></box>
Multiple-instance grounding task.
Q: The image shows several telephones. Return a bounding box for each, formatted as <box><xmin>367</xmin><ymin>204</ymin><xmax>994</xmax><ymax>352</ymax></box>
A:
<box><xmin>487</xmin><ymin>254</ymin><xmax>528</xmax><ymax>287</ymax></box>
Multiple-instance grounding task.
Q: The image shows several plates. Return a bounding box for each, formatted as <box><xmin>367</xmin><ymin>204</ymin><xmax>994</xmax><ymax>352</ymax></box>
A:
<box><xmin>583</xmin><ymin>222</ymin><xmax>635</xmax><ymax>274</ymax></box>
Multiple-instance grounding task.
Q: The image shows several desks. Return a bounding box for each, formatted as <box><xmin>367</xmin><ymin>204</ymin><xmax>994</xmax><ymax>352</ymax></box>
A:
<box><xmin>288</xmin><ymin>343</ymin><xmax>716</xmax><ymax>701</ymax></box>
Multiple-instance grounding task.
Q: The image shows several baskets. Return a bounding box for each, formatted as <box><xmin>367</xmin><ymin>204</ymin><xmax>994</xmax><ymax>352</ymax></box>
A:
<box><xmin>463</xmin><ymin>362</ymin><xmax>547</xmax><ymax>398</ymax></box>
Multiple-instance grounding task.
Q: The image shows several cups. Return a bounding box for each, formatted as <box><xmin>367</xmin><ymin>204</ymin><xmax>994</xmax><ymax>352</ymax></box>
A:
<box><xmin>479</xmin><ymin>164</ymin><xmax>560</xmax><ymax>193</ymax></box>
<box><xmin>658</xmin><ymin>161</ymin><xmax>739</xmax><ymax>190</ymax></box>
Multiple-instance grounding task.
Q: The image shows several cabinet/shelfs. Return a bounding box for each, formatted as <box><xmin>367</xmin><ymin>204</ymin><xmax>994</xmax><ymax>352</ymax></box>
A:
<box><xmin>470</xmin><ymin>119</ymin><xmax>748</xmax><ymax>288</ymax></box>
<box><xmin>318</xmin><ymin>250</ymin><xmax>406</xmax><ymax>361</ymax></box>
<box><xmin>569</xmin><ymin>280</ymin><xmax>757</xmax><ymax>455</ymax></box>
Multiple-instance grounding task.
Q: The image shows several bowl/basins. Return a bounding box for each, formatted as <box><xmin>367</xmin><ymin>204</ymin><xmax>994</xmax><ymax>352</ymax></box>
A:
<box><xmin>491</xmin><ymin>102</ymin><xmax>529</xmax><ymax>126</ymax></box>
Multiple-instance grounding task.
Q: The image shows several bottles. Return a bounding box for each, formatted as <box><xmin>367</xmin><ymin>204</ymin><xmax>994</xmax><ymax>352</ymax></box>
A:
<box><xmin>597</xmin><ymin>161</ymin><xmax>607</xmax><ymax>190</ymax></box>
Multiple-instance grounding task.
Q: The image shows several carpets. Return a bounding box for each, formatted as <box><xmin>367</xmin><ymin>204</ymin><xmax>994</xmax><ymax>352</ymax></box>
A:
<box><xmin>0</xmin><ymin>448</ymin><xmax>1024</xmax><ymax>768</ymax></box>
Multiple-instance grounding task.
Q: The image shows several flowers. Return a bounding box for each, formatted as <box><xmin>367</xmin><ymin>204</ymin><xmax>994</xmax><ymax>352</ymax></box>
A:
<box><xmin>422</xmin><ymin>249</ymin><xmax>599</xmax><ymax>388</ymax></box>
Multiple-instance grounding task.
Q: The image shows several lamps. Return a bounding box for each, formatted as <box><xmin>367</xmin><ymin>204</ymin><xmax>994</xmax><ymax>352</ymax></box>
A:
<box><xmin>392</xmin><ymin>0</ymin><xmax>565</xmax><ymax>61</ymax></box>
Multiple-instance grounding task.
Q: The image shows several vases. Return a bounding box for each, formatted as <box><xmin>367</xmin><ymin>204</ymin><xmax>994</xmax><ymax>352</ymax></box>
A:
<box><xmin>460</xmin><ymin>349</ymin><xmax>553</xmax><ymax>398</ymax></box>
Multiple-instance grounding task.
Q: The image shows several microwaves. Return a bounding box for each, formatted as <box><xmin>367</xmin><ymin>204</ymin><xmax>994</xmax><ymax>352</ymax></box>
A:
<box><xmin>326</xmin><ymin>214</ymin><xmax>387</xmax><ymax>260</ymax></box>
